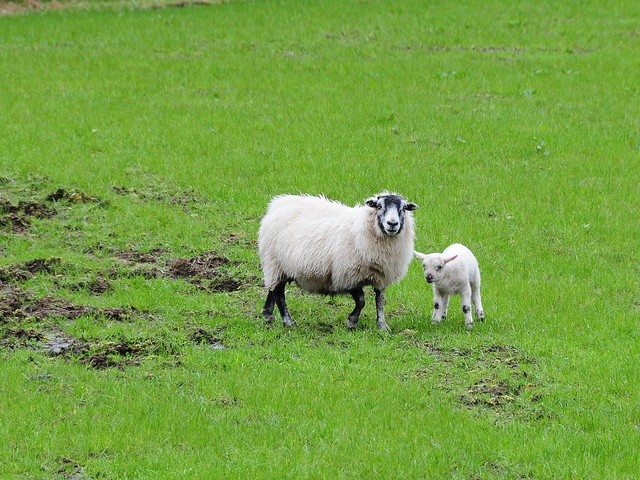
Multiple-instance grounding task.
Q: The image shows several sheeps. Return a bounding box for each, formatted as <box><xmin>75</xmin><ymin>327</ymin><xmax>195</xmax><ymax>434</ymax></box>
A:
<box><xmin>413</xmin><ymin>241</ymin><xmax>485</xmax><ymax>333</ymax></box>
<box><xmin>255</xmin><ymin>189</ymin><xmax>419</xmax><ymax>334</ymax></box>
